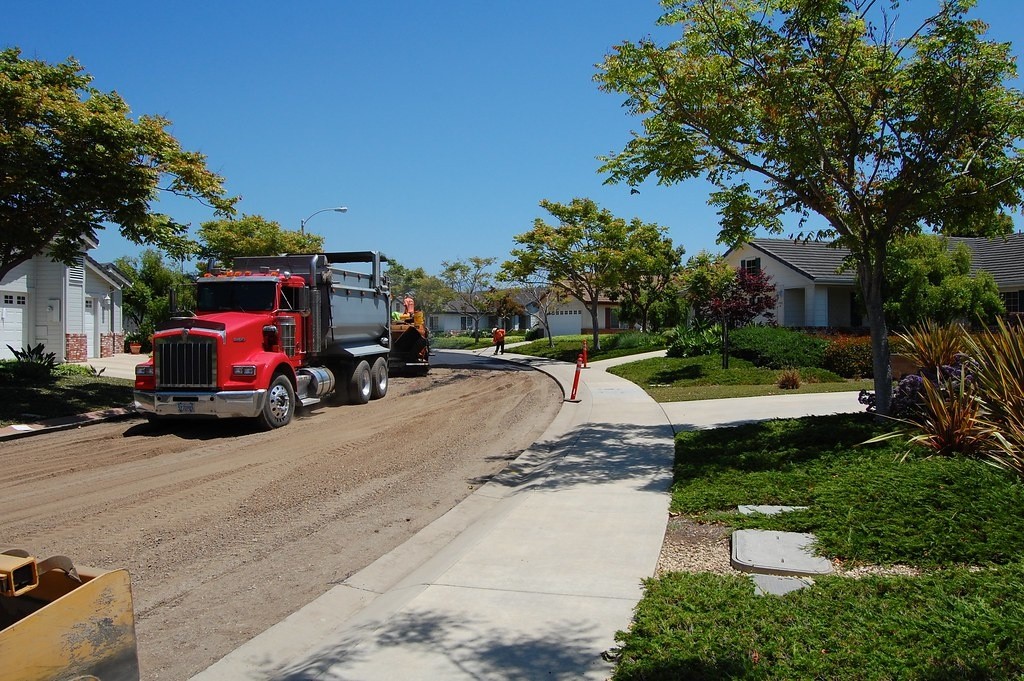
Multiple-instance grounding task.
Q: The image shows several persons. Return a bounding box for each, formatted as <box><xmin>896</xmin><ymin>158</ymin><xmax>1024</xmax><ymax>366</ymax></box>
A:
<box><xmin>491</xmin><ymin>328</ymin><xmax>505</xmax><ymax>355</ymax></box>
<box><xmin>403</xmin><ymin>293</ymin><xmax>414</xmax><ymax>318</ymax></box>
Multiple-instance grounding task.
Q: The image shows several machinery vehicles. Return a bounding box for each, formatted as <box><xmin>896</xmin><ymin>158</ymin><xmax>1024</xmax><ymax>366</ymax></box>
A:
<box><xmin>388</xmin><ymin>311</ymin><xmax>432</xmax><ymax>378</ymax></box>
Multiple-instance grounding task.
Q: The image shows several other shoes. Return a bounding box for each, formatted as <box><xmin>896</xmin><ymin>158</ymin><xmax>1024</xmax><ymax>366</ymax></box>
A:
<box><xmin>494</xmin><ymin>352</ymin><xmax>497</xmax><ymax>355</ymax></box>
<box><xmin>501</xmin><ymin>352</ymin><xmax>503</xmax><ymax>355</ymax></box>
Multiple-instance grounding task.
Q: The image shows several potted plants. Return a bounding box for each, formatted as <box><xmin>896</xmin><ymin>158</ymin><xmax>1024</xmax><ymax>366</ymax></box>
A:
<box><xmin>130</xmin><ymin>343</ymin><xmax>142</xmax><ymax>354</ymax></box>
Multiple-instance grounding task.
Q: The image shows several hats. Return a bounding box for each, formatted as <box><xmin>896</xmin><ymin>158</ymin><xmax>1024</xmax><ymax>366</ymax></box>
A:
<box><xmin>492</xmin><ymin>327</ymin><xmax>497</xmax><ymax>333</ymax></box>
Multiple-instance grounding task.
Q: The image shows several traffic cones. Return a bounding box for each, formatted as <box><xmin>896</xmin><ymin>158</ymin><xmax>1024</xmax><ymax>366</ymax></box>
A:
<box><xmin>564</xmin><ymin>353</ymin><xmax>583</xmax><ymax>403</ymax></box>
<box><xmin>581</xmin><ymin>339</ymin><xmax>591</xmax><ymax>369</ymax></box>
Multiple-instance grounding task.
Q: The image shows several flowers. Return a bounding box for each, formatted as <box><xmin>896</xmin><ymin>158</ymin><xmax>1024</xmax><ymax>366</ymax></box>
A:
<box><xmin>123</xmin><ymin>331</ymin><xmax>146</xmax><ymax>343</ymax></box>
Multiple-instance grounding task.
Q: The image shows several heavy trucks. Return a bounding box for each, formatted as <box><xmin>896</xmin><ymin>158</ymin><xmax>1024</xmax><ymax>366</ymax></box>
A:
<box><xmin>133</xmin><ymin>250</ymin><xmax>392</xmax><ymax>430</ymax></box>
